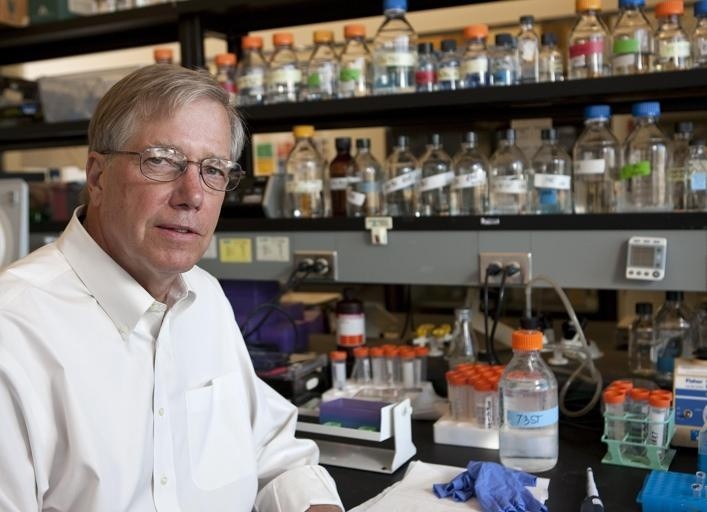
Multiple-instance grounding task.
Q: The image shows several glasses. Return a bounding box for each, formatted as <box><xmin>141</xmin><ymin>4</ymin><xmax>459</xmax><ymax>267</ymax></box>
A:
<box><xmin>98</xmin><ymin>145</ymin><xmax>242</xmax><ymax>192</ymax></box>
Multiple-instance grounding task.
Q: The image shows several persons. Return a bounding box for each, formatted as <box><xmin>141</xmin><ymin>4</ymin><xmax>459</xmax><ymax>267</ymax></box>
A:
<box><xmin>0</xmin><ymin>65</ymin><xmax>349</xmax><ymax>512</ymax></box>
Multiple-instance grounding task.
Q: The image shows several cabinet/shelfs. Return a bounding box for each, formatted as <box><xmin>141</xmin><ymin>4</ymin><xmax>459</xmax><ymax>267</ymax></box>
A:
<box><xmin>0</xmin><ymin>0</ymin><xmax>707</xmax><ymax>292</ymax></box>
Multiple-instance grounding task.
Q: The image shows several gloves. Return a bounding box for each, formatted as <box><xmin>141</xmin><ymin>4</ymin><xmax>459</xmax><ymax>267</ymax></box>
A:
<box><xmin>434</xmin><ymin>460</ymin><xmax>547</xmax><ymax>511</ymax></box>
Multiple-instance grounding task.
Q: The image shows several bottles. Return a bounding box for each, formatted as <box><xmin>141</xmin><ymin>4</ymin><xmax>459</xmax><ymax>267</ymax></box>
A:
<box><xmin>495</xmin><ymin>130</ymin><xmax>532</xmax><ymax>213</ymax></box>
<box><xmin>465</xmin><ymin>22</ymin><xmax>493</xmax><ymax>87</ymax></box>
<box><xmin>238</xmin><ymin>36</ymin><xmax>270</xmax><ymax>105</ymax></box>
<box><xmin>533</xmin><ymin>129</ymin><xmax>574</xmax><ymax>214</ymax></box>
<box><xmin>154</xmin><ymin>48</ymin><xmax>174</xmax><ymax>65</ymax></box>
<box><xmin>656</xmin><ymin>0</ymin><xmax>693</xmax><ymax>74</ymax></box>
<box><xmin>516</xmin><ymin>16</ymin><xmax>544</xmax><ymax>82</ymax></box>
<box><xmin>566</xmin><ymin>1</ymin><xmax>613</xmax><ymax>81</ymax></box>
<box><xmin>575</xmin><ymin>105</ymin><xmax>628</xmax><ymax>214</ymax></box>
<box><xmin>497</xmin><ymin>334</ymin><xmax>557</xmax><ymax>472</ymax></box>
<box><xmin>455</xmin><ymin>131</ymin><xmax>493</xmax><ymax>216</ymax></box>
<box><xmin>493</xmin><ymin>32</ymin><xmax>521</xmax><ymax>83</ymax></box>
<box><xmin>383</xmin><ymin>137</ymin><xmax>417</xmax><ymax>216</ymax></box>
<box><xmin>693</xmin><ymin>0</ymin><xmax>707</xmax><ymax>68</ymax></box>
<box><xmin>270</xmin><ymin>32</ymin><xmax>305</xmax><ymax>102</ymax></box>
<box><xmin>49</xmin><ymin>168</ymin><xmax>68</xmax><ymax>220</ymax></box>
<box><xmin>418</xmin><ymin>42</ymin><xmax>440</xmax><ymax>89</ymax></box>
<box><xmin>418</xmin><ymin>136</ymin><xmax>455</xmax><ymax>217</ymax></box>
<box><xmin>602</xmin><ymin>380</ymin><xmax>673</xmax><ymax>460</ymax></box>
<box><xmin>348</xmin><ymin>138</ymin><xmax>383</xmax><ymax>215</ymax></box>
<box><xmin>305</xmin><ymin>30</ymin><xmax>339</xmax><ymax>100</ymax></box>
<box><xmin>626</xmin><ymin>102</ymin><xmax>674</xmax><ymax>212</ymax></box>
<box><xmin>26</xmin><ymin>173</ymin><xmax>49</xmax><ymax>233</ymax></box>
<box><xmin>445</xmin><ymin>308</ymin><xmax>477</xmax><ymax>372</ymax></box>
<box><xmin>614</xmin><ymin>0</ymin><xmax>656</xmax><ymax>72</ymax></box>
<box><xmin>697</xmin><ymin>421</ymin><xmax>706</xmax><ymax>475</ymax></box>
<box><xmin>674</xmin><ymin>100</ymin><xmax>707</xmax><ymax>219</ymax></box>
<box><xmin>280</xmin><ymin>126</ymin><xmax>331</xmax><ymax>217</ymax></box>
<box><xmin>445</xmin><ymin>360</ymin><xmax>507</xmax><ymax>430</ymax></box>
<box><xmin>339</xmin><ymin>24</ymin><xmax>373</xmax><ymax>98</ymax></box>
<box><xmin>373</xmin><ymin>1</ymin><xmax>418</xmax><ymax>91</ymax></box>
<box><xmin>539</xmin><ymin>33</ymin><xmax>566</xmax><ymax>81</ymax></box>
<box><xmin>335</xmin><ymin>298</ymin><xmax>368</xmax><ymax>363</ymax></box>
<box><xmin>331</xmin><ymin>138</ymin><xmax>360</xmax><ymax>217</ymax></box>
<box><xmin>440</xmin><ymin>39</ymin><xmax>465</xmax><ymax>90</ymax></box>
<box><xmin>579</xmin><ymin>466</ymin><xmax>604</xmax><ymax>511</ymax></box>
<box><xmin>629</xmin><ymin>289</ymin><xmax>706</xmax><ymax>385</ymax></box>
<box><xmin>211</xmin><ymin>53</ymin><xmax>238</xmax><ymax>104</ymax></box>
<box><xmin>328</xmin><ymin>322</ymin><xmax>450</xmax><ymax>392</ymax></box>
